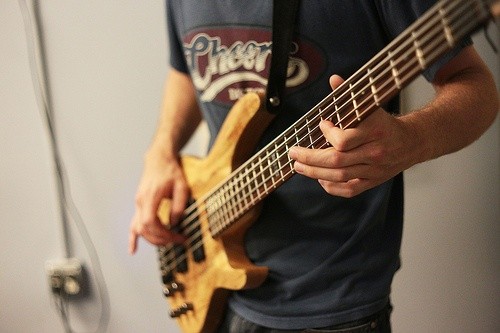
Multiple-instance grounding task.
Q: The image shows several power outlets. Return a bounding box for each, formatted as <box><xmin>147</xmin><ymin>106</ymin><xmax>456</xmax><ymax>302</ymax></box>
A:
<box><xmin>47</xmin><ymin>263</ymin><xmax>84</xmax><ymax>297</ymax></box>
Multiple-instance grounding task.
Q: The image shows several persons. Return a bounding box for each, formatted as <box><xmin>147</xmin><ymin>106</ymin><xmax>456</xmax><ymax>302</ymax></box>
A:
<box><xmin>130</xmin><ymin>0</ymin><xmax>500</xmax><ymax>333</ymax></box>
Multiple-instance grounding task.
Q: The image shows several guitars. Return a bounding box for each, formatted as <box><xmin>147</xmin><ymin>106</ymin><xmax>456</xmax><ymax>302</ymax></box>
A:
<box><xmin>158</xmin><ymin>0</ymin><xmax>500</xmax><ymax>333</ymax></box>
